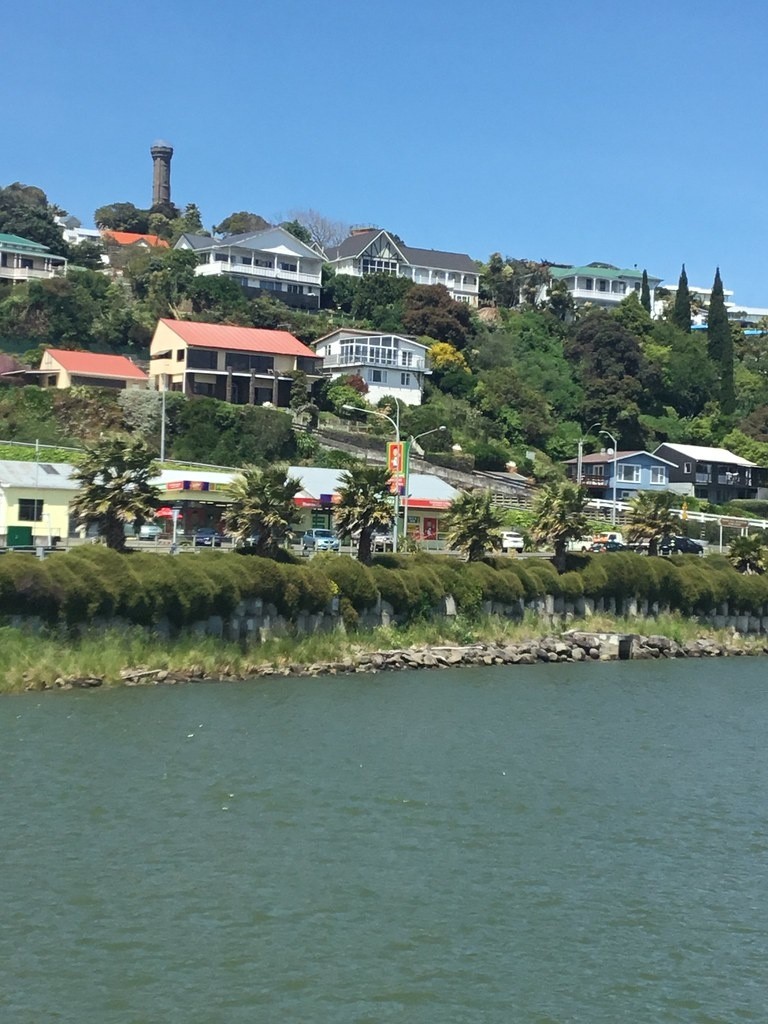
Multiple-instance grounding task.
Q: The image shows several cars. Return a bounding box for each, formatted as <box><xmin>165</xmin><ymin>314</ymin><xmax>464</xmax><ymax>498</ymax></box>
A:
<box><xmin>121</xmin><ymin>518</ymin><xmax>163</xmax><ymax>540</ymax></box>
<box><xmin>195</xmin><ymin>528</ymin><xmax>221</xmax><ymax>547</ymax></box>
<box><xmin>498</xmin><ymin>531</ymin><xmax>526</xmax><ymax>553</ymax></box>
<box><xmin>304</xmin><ymin>528</ymin><xmax>341</xmax><ymax>553</ymax></box>
<box><xmin>659</xmin><ymin>537</ymin><xmax>704</xmax><ymax>558</ymax></box>
<box><xmin>565</xmin><ymin>529</ymin><xmax>651</xmax><ymax>558</ymax></box>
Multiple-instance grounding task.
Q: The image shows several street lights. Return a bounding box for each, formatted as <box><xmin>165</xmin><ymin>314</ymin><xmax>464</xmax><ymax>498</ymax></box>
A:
<box><xmin>344</xmin><ymin>404</ymin><xmax>399</xmax><ymax>554</ymax></box>
<box><xmin>577</xmin><ymin>423</ymin><xmax>602</xmax><ymax>489</ymax></box>
<box><xmin>403</xmin><ymin>426</ymin><xmax>447</xmax><ymax>538</ymax></box>
<box><xmin>598</xmin><ymin>430</ymin><xmax>617</xmax><ymax>526</ymax></box>
